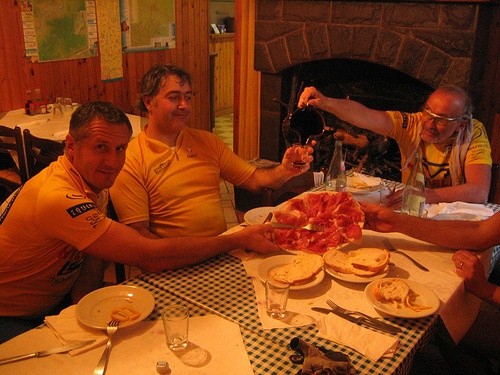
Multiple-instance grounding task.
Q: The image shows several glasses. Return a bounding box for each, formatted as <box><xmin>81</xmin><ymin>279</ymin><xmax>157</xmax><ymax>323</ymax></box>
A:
<box><xmin>422</xmin><ymin>107</ymin><xmax>464</xmax><ymax>127</ymax></box>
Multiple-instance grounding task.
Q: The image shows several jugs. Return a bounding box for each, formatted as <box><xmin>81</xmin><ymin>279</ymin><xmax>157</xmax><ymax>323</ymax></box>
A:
<box><xmin>282</xmin><ymin>104</ymin><xmax>325</xmax><ymax>146</ymax></box>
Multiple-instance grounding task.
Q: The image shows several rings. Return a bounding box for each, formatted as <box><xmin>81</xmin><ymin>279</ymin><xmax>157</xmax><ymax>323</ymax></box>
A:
<box><xmin>460</xmin><ymin>262</ymin><xmax>463</xmax><ymax>266</ymax></box>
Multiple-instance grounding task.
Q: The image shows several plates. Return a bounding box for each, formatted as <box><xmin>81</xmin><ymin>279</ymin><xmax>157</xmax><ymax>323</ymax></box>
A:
<box><xmin>256</xmin><ymin>255</ymin><xmax>324</xmax><ymax>290</ymax></box>
<box><xmin>75</xmin><ymin>285</ymin><xmax>155</xmax><ymax>329</ymax></box>
<box><xmin>244</xmin><ymin>207</ymin><xmax>275</xmax><ymax>225</ymax></box>
<box><xmin>323</xmin><ymin>250</ymin><xmax>390</xmax><ymax>283</ymax></box>
<box><xmin>364</xmin><ymin>278</ymin><xmax>440</xmax><ymax>318</ymax></box>
<box><xmin>271</xmin><ymin>209</ymin><xmax>364</xmax><ymax>255</ymax></box>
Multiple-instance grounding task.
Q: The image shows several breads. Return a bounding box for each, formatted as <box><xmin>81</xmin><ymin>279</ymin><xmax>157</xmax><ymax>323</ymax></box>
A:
<box><xmin>373</xmin><ymin>278</ymin><xmax>410</xmax><ymax>303</ymax></box>
<box><xmin>273</xmin><ymin>254</ymin><xmax>324</xmax><ymax>285</ymax></box>
<box><xmin>323</xmin><ymin>248</ymin><xmax>389</xmax><ymax>277</ymax></box>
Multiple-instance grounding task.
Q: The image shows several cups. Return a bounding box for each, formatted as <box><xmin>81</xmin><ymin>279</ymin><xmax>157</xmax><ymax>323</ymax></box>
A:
<box><xmin>380</xmin><ymin>182</ymin><xmax>395</xmax><ymax>207</ymax></box>
<box><xmin>423</xmin><ymin>208</ymin><xmax>428</xmax><ymax>219</ymax></box>
<box><xmin>53</xmin><ymin>97</ymin><xmax>73</xmax><ymax>120</ymax></box>
<box><xmin>162</xmin><ymin>306</ymin><xmax>190</xmax><ymax>352</ymax></box>
<box><xmin>265</xmin><ymin>278</ymin><xmax>290</xmax><ymax>319</ymax></box>
<box><xmin>291</xmin><ymin>144</ymin><xmax>308</xmax><ymax>170</ymax></box>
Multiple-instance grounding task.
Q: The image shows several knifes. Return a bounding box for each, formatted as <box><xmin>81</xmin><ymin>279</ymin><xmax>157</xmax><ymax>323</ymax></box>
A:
<box><xmin>0</xmin><ymin>339</ymin><xmax>96</xmax><ymax>365</ymax></box>
<box><xmin>328</xmin><ymin>310</ymin><xmax>397</xmax><ymax>335</ymax></box>
<box><xmin>246</xmin><ymin>213</ymin><xmax>272</xmax><ymax>253</ymax></box>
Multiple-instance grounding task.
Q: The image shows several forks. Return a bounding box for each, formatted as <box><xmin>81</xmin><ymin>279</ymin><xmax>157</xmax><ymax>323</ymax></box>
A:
<box><xmin>239</xmin><ymin>223</ymin><xmax>322</xmax><ymax>231</ymax></box>
<box><xmin>326</xmin><ymin>299</ymin><xmax>403</xmax><ymax>332</ymax></box>
<box><xmin>382</xmin><ymin>237</ymin><xmax>430</xmax><ymax>272</ymax></box>
<box><xmin>93</xmin><ymin>321</ymin><xmax>120</xmax><ymax>375</ymax></box>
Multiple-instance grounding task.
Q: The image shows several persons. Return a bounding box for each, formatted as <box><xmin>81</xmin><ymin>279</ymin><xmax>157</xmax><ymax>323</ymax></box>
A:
<box><xmin>297</xmin><ymin>85</ymin><xmax>492</xmax><ymax>210</ymax></box>
<box><xmin>0</xmin><ymin>101</ymin><xmax>282</xmax><ymax>335</ymax></box>
<box><xmin>360</xmin><ymin>206</ymin><xmax>500</xmax><ymax>375</ymax></box>
<box><xmin>109</xmin><ymin>62</ymin><xmax>314</xmax><ymax>240</ymax></box>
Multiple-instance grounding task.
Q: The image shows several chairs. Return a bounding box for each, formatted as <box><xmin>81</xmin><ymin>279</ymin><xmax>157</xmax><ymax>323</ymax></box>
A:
<box><xmin>0</xmin><ymin>125</ymin><xmax>127</xmax><ymax>284</ymax></box>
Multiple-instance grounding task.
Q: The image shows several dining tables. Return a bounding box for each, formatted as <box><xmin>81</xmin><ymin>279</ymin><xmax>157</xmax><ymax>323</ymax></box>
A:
<box><xmin>0</xmin><ymin>169</ymin><xmax>500</xmax><ymax>375</ymax></box>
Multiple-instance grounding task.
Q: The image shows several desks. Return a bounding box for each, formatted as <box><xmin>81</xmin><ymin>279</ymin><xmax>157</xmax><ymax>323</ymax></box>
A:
<box><xmin>0</xmin><ymin>104</ymin><xmax>149</xmax><ymax>174</ymax></box>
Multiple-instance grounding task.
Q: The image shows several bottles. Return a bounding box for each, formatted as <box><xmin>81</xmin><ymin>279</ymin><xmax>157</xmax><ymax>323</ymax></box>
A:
<box><xmin>25</xmin><ymin>90</ymin><xmax>33</xmax><ymax>115</ymax></box>
<box><xmin>40</xmin><ymin>102</ymin><xmax>48</xmax><ymax>114</ymax></box>
<box><xmin>401</xmin><ymin>152</ymin><xmax>425</xmax><ymax>219</ymax></box>
<box><xmin>34</xmin><ymin>89</ymin><xmax>43</xmax><ymax>114</ymax></box>
<box><xmin>325</xmin><ymin>141</ymin><xmax>347</xmax><ymax>193</ymax></box>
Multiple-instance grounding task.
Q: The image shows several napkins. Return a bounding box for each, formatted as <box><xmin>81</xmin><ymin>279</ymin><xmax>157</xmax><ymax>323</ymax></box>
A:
<box><xmin>353</xmin><ymin>172</ymin><xmax>381</xmax><ymax>189</ymax></box>
<box><xmin>42</xmin><ymin>315</ymin><xmax>109</xmax><ymax>357</ymax></box>
<box><xmin>315</xmin><ymin>312</ymin><xmax>400</xmax><ymax>363</ymax></box>
<box><xmin>436</xmin><ymin>201</ymin><xmax>494</xmax><ymax>217</ymax></box>
